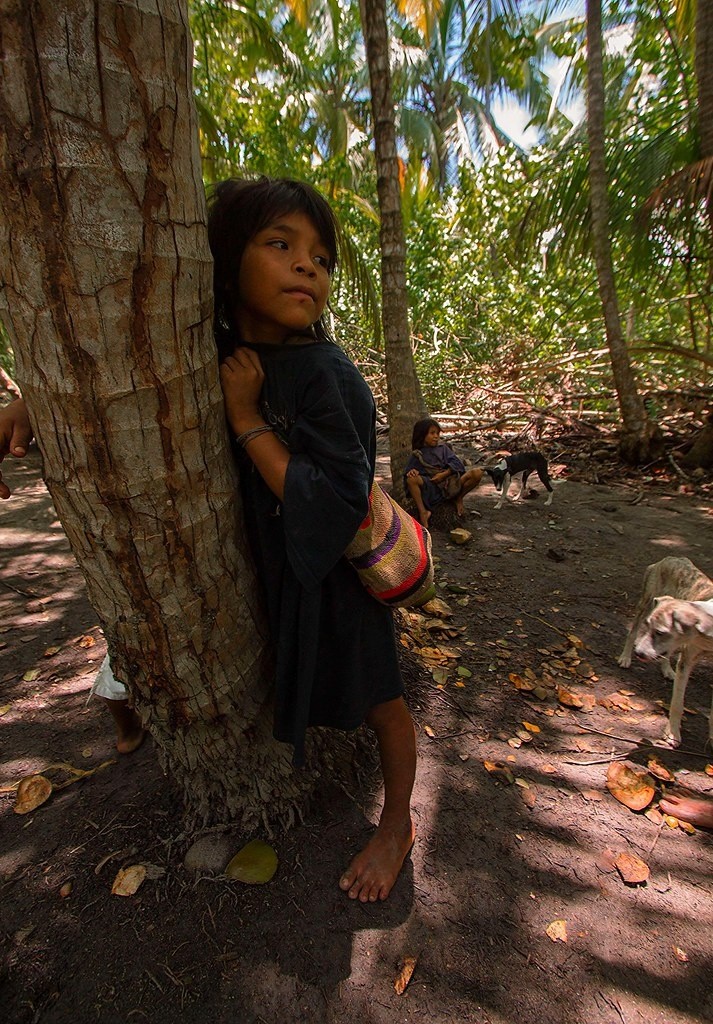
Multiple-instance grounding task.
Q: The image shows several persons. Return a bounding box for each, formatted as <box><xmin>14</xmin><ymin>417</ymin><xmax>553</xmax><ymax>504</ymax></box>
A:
<box><xmin>0</xmin><ymin>178</ymin><xmax>416</xmax><ymax>904</ymax></box>
<box><xmin>402</xmin><ymin>419</ymin><xmax>482</xmax><ymax>529</ymax></box>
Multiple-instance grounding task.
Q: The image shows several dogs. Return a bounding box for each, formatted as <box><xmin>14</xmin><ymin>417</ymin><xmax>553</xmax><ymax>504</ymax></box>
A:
<box><xmin>483</xmin><ymin>453</ymin><xmax>554</xmax><ymax>510</ymax></box>
<box><xmin>616</xmin><ymin>554</ymin><xmax>713</xmax><ymax>747</ymax></box>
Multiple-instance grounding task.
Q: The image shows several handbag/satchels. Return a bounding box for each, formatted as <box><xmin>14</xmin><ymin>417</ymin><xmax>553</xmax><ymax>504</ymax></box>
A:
<box><xmin>413</xmin><ymin>449</ymin><xmax>462</xmax><ymax>499</ymax></box>
<box><xmin>343</xmin><ymin>478</ymin><xmax>442</xmax><ymax>608</ymax></box>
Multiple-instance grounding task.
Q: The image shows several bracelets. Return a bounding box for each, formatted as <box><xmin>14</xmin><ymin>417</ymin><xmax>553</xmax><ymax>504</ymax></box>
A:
<box><xmin>234</xmin><ymin>424</ymin><xmax>274</xmax><ymax>446</ymax></box>
